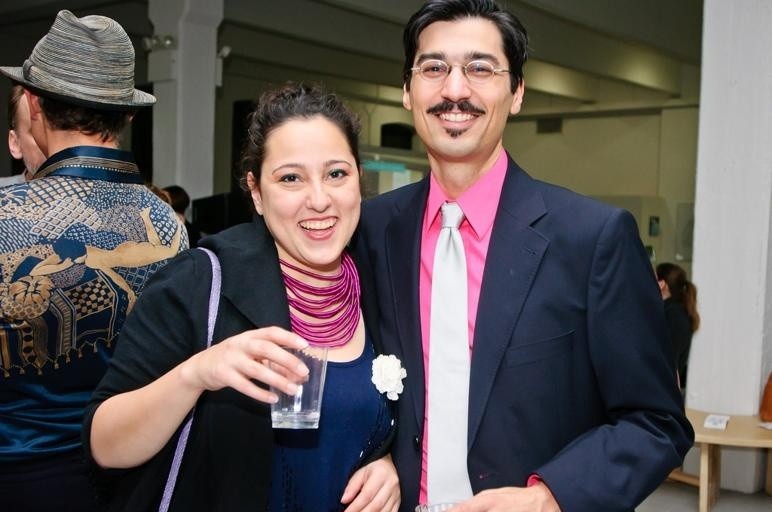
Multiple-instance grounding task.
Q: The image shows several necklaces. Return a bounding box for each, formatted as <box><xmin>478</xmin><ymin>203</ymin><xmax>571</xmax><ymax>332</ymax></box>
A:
<box><xmin>279</xmin><ymin>251</ymin><xmax>362</xmax><ymax>351</ymax></box>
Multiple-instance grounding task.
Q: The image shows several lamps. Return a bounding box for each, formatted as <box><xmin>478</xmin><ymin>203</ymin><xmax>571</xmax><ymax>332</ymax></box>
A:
<box><xmin>216</xmin><ymin>46</ymin><xmax>232</xmax><ymax>58</ymax></box>
<box><xmin>143</xmin><ymin>36</ymin><xmax>175</xmax><ymax>50</ymax></box>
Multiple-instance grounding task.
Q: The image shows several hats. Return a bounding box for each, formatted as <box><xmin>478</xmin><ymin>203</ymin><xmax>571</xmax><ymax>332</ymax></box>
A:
<box><xmin>0</xmin><ymin>9</ymin><xmax>157</xmax><ymax>111</ymax></box>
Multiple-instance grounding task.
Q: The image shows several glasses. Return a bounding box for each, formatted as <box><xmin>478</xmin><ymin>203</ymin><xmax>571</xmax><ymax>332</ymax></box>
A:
<box><xmin>411</xmin><ymin>59</ymin><xmax>512</xmax><ymax>83</ymax></box>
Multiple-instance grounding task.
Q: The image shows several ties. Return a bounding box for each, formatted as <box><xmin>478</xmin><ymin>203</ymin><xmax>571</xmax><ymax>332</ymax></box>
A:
<box><xmin>428</xmin><ymin>201</ymin><xmax>475</xmax><ymax>512</ymax></box>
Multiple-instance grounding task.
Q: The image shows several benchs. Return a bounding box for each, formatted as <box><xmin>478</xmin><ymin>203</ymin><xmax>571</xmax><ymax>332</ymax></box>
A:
<box><xmin>665</xmin><ymin>406</ymin><xmax>772</xmax><ymax>512</ymax></box>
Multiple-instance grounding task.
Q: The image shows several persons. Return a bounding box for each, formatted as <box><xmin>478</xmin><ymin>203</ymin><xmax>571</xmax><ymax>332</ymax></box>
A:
<box><xmin>1</xmin><ymin>84</ymin><xmax>52</xmax><ymax>191</ymax></box>
<box><xmin>80</xmin><ymin>77</ymin><xmax>408</xmax><ymax>512</ymax></box>
<box><xmin>0</xmin><ymin>7</ymin><xmax>193</xmax><ymax>511</ymax></box>
<box><xmin>649</xmin><ymin>263</ymin><xmax>700</xmax><ymax>395</ymax></box>
<box><xmin>160</xmin><ymin>185</ymin><xmax>202</xmax><ymax>250</ymax></box>
<box><xmin>336</xmin><ymin>0</ymin><xmax>701</xmax><ymax>511</ymax></box>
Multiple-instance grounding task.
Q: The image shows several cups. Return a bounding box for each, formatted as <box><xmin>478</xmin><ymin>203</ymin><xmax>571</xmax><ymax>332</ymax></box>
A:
<box><xmin>414</xmin><ymin>502</ymin><xmax>462</xmax><ymax>512</ymax></box>
<box><xmin>267</xmin><ymin>344</ymin><xmax>331</xmax><ymax>430</ymax></box>
